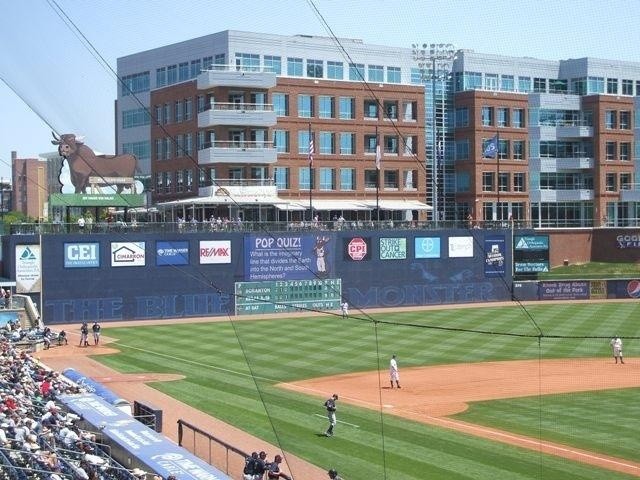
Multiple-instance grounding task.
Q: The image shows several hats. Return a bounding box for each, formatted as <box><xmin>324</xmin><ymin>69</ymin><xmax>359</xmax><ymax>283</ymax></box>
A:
<box><xmin>275</xmin><ymin>455</ymin><xmax>283</xmax><ymax>460</ymax></box>
<box><xmin>0</xmin><ymin>315</ymin><xmax>102</xmax><ymax>480</ymax></box>
<box><xmin>252</xmin><ymin>451</ymin><xmax>268</xmax><ymax>456</ymax></box>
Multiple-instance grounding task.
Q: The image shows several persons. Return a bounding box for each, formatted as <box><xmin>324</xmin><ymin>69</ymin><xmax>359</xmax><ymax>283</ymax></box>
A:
<box><xmin>610</xmin><ymin>334</ymin><xmax>626</xmax><ymax>364</ymax></box>
<box><xmin>0</xmin><ymin>286</ymin><xmax>179</xmax><ymax>479</ymax></box>
<box><xmin>242</xmin><ymin>452</ymin><xmax>259</xmax><ymax>480</ymax></box>
<box><xmin>254</xmin><ymin>451</ymin><xmax>271</xmax><ymax>479</ymax></box>
<box><xmin>267</xmin><ymin>454</ymin><xmax>285</xmax><ymax>480</ymax></box>
<box><xmin>311</xmin><ymin>236</ymin><xmax>329</xmax><ymax>278</ymax></box>
<box><xmin>322</xmin><ymin>394</ymin><xmax>339</xmax><ymax>437</ymax></box>
<box><xmin>326</xmin><ymin>469</ymin><xmax>342</xmax><ymax>480</ymax></box>
<box><xmin>340</xmin><ymin>300</ymin><xmax>350</xmax><ymax>319</ymax></box>
<box><xmin>388</xmin><ymin>353</ymin><xmax>402</xmax><ymax>389</ymax></box>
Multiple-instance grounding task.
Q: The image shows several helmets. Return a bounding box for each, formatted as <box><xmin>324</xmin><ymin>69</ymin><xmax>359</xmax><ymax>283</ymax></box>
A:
<box><xmin>333</xmin><ymin>394</ymin><xmax>338</xmax><ymax>400</ymax></box>
<box><xmin>328</xmin><ymin>469</ymin><xmax>337</xmax><ymax>477</ymax></box>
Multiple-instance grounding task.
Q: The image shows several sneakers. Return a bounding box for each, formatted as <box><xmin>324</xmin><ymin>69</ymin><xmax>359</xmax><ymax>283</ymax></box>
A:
<box><xmin>325</xmin><ymin>431</ymin><xmax>334</xmax><ymax>437</ymax></box>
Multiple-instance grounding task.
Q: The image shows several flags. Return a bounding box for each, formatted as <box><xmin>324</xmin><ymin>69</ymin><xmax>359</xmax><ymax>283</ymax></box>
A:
<box><xmin>480</xmin><ymin>132</ymin><xmax>499</xmax><ymax>160</ymax></box>
<box><xmin>375</xmin><ymin>131</ymin><xmax>383</xmax><ymax>172</ymax></box>
<box><xmin>307</xmin><ymin>125</ymin><xmax>315</xmax><ymax>168</ymax></box>
